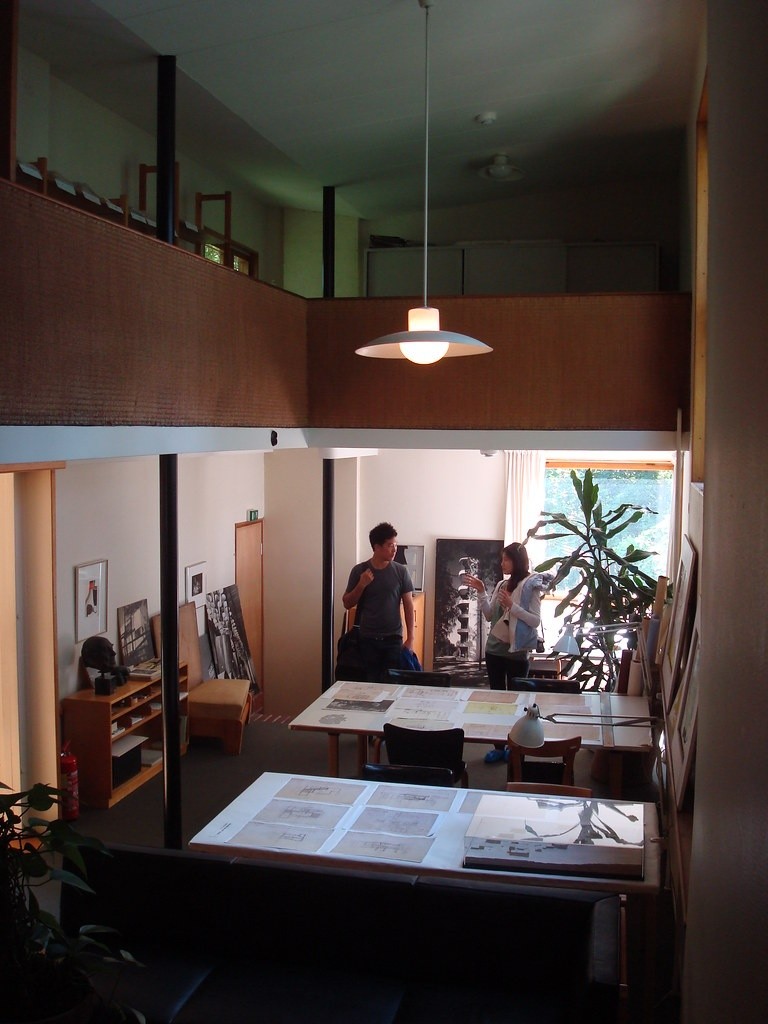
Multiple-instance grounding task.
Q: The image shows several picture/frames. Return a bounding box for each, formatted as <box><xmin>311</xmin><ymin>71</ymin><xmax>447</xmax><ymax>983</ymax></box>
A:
<box><xmin>663</xmin><ymin>533</ymin><xmax>695</xmax><ymax>714</ymax></box>
<box><xmin>185</xmin><ymin>562</ymin><xmax>209</xmax><ymax>609</ymax></box>
<box><xmin>76</xmin><ymin>560</ymin><xmax>108</xmax><ymax>644</ymax></box>
<box><xmin>394</xmin><ymin>544</ymin><xmax>425</xmax><ymax>592</ymax></box>
<box><xmin>672</xmin><ymin>595</ymin><xmax>702</xmax><ymax>807</ymax></box>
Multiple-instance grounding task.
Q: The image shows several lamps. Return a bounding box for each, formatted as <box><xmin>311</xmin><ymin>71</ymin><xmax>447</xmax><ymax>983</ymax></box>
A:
<box><xmin>354</xmin><ymin>0</ymin><xmax>492</xmax><ymax>363</ymax></box>
<box><xmin>554</xmin><ymin>622</ymin><xmax>642</xmax><ymax>655</ymax></box>
<box><xmin>510</xmin><ymin>705</ymin><xmax>664</xmax><ymax>750</ymax></box>
<box><xmin>479</xmin><ymin>154</ymin><xmax>528</xmax><ymax>183</ymax></box>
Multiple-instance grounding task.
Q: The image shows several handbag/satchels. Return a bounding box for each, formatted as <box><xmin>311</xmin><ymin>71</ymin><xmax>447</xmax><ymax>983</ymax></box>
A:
<box><xmin>335</xmin><ymin>627</ymin><xmax>370</xmax><ymax>682</ymax></box>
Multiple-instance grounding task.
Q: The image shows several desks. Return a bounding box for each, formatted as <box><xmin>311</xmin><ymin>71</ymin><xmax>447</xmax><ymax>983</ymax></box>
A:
<box><xmin>287</xmin><ymin>680</ymin><xmax>654</xmax><ymax>801</ymax></box>
<box><xmin>187</xmin><ymin>768</ymin><xmax>664</xmax><ymax>1013</ymax></box>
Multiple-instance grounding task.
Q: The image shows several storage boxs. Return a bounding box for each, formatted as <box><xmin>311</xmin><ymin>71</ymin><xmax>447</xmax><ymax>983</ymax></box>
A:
<box><xmin>111</xmin><ymin>745</ymin><xmax>141</xmax><ymax>790</ymax></box>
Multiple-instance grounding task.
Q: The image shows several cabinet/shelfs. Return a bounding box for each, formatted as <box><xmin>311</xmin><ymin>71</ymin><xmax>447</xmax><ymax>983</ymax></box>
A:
<box><xmin>17</xmin><ymin>145</ymin><xmax>258</xmax><ymax>279</ymax></box>
<box><xmin>348</xmin><ymin>592</ymin><xmax>424</xmax><ymax>670</ymax></box>
<box><xmin>62</xmin><ymin>660</ymin><xmax>193</xmax><ymax>811</ymax></box>
<box><xmin>361</xmin><ymin>240</ymin><xmax>664</xmax><ymax>291</ymax></box>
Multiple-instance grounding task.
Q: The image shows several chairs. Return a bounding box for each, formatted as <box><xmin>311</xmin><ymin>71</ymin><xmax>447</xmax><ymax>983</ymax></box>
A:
<box><xmin>367</xmin><ymin>671</ymin><xmax>471</xmax><ymax>787</ymax></box>
<box><xmin>508</xmin><ymin>738</ymin><xmax>593</xmax><ymax>798</ymax></box>
<box><xmin>510</xmin><ymin>656</ymin><xmax>581</xmax><ymax>695</ymax></box>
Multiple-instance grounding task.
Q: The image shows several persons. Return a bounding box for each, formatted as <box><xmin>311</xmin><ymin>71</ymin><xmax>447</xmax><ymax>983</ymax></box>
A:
<box><xmin>461</xmin><ymin>543</ymin><xmax>554</xmax><ymax>764</ymax></box>
<box><xmin>341</xmin><ymin>522</ymin><xmax>414</xmax><ymax>742</ymax></box>
<box><xmin>80</xmin><ymin>635</ymin><xmax>117</xmax><ymax>672</ymax></box>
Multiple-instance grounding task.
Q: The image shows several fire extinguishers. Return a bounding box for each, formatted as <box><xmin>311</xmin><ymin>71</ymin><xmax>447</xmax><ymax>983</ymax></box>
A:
<box><xmin>60</xmin><ymin>740</ymin><xmax>79</xmax><ymax>822</ymax></box>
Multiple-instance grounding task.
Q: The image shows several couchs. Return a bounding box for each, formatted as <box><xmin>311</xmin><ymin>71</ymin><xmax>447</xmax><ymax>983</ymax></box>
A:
<box><xmin>60</xmin><ymin>843</ymin><xmax>632</xmax><ymax>1024</ymax></box>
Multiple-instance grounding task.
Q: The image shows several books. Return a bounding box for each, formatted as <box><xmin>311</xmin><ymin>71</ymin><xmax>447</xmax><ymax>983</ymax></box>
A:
<box><xmin>129</xmin><ymin>655</ymin><xmax>161</xmax><ymax>681</ymax></box>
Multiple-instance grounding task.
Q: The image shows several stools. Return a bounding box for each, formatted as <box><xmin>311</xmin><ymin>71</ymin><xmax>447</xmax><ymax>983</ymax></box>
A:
<box><xmin>187</xmin><ymin>679</ymin><xmax>252</xmax><ymax>753</ymax></box>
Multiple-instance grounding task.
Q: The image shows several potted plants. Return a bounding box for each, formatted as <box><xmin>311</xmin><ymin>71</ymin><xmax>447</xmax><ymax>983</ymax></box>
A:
<box><xmin>0</xmin><ymin>779</ymin><xmax>149</xmax><ymax>1024</ymax></box>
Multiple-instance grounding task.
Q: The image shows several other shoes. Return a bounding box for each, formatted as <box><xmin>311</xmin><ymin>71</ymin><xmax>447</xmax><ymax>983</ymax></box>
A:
<box><xmin>484</xmin><ymin>748</ymin><xmax>510</xmax><ymax>764</ymax></box>
